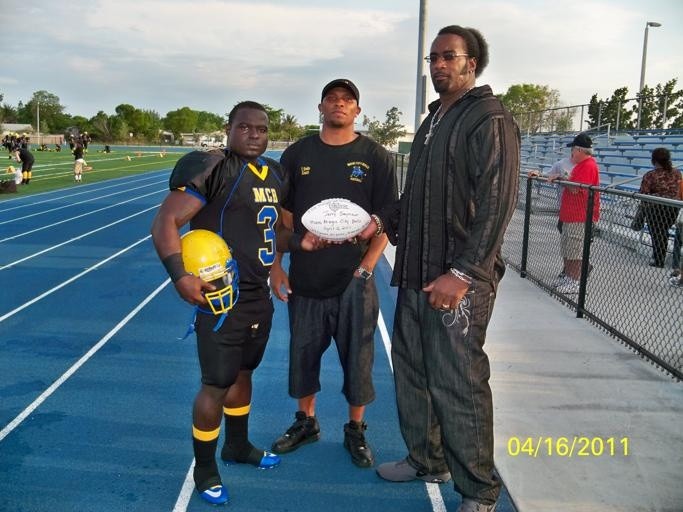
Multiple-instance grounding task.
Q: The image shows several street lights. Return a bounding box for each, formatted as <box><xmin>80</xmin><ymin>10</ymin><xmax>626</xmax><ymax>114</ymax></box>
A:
<box><xmin>634</xmin><ymin>20</ymin><xmax>661</xmax><ymax>135</ymax></box>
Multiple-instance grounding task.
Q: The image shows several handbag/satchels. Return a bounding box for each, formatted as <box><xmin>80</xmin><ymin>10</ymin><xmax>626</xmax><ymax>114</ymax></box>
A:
<box><xmin>632</xmin><ymin>204</ymin><xmax>645</xmax><ymax>231</ymax></box>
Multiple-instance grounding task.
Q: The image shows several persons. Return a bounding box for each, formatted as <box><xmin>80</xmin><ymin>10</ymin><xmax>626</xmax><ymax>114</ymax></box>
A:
<box><xmin>667</xmin><ymin>205</ymin><xmax>683</xmax><ymax>288</ymax></box>
<box><xmin>638</xmin><ymin>147</ymin><xmax>682</xmax><ymax>268</ymax></box>
<box><xmin>154</xmin><ymin>103</ymin><xmax>331</xmax><ymax>506</ymax></box>
<box><xmin>528</xmin><ymin>140</ymin><xmax>593</xmax><ymax>281</ymax></box>
<box><xmin>268</xmin><ymin>79</ymin><xmax>399</xmax><ymax>468</ymax></box>
<box><xmin>331</xmin><ymin>26</ymin><xmax>519</xmax><ymax>511</ymax></box>
<box><xmin>0</xmin><ymin>126</ymin><xmax>111</xmax><ymax>186</ymax></box>
<box><xmin>556</xmin><ymin>133</ymin><xmax>600</xmax><ymax>295</ymax></box>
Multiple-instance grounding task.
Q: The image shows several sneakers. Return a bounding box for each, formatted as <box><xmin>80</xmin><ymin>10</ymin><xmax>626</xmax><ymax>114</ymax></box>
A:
<box><xmin>551</xmin><ymin>277</ymin><xmax>581</xmax><ymax>294</ymax></box>
<box><xmin>193</xmin><ymin>463</ymin><xmax>229</xmax><ymax>505</ymax></box>
<box><xmin>343</xmin><ymin>421</ymin><xmax>374</xmax><ymax>467</ymax></box>
<box><xmin>222</xmin><ymin>445</ymin><xmax>280</xmax><ymax>469</ymax></box>
<box><xmin>457</xmin><ymin>497</ymin><xmax>496</xmax><ymax>512</ymax></box>
<box><xmin>588</xmin><ymin>265</ymin><xmax>594</xmax><ymax>278</ymax></box>
<box><xmin>272</xmin><ymin>412</ymin><xmax>321</xmax><ymax>453</ymax></box>
<box><xmin>378</xmin><ymin>460</ymin><xmax>451</xmax><ymax>483</ymax></box>
<box><xmin>670</xmin><ymin>276</ymin><xmax>683</xmax><ymax>287</ymax></box>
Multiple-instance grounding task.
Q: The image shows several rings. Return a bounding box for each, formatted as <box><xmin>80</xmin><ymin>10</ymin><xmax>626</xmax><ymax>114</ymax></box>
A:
<box><xmin>442</xmin><ymin>304</ymin><xmax>450</xmax><ymax>308</ymax></box>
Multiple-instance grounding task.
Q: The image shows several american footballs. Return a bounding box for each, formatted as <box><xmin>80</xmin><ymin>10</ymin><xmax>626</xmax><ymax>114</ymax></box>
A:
<box><xmin>301</xmin><ymin>197</ymin><xmax>372</xmax><ymax>241</ymax></box>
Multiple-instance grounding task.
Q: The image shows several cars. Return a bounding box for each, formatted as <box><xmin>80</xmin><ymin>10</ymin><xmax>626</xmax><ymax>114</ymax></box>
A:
<box><xmin>182</xmin><ymin>138</ymin><xmax>195</xmax><ymax>146</ymax></box>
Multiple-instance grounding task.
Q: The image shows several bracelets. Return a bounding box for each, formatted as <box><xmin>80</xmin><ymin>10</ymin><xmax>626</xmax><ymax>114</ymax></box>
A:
<box><xmin>288</xmin><ymin>233</ymin><xmax>302</xmax><ymax>251</ymax></box>
<box><xmin>162</xmin><ymin>254</ymin><xmax>188</xmax><ymax>282</ymax></box>
<box><xmin>371</xmin><ymin>213</ymin><xmax>384</xmax><ymax>237</ymax></box>
<box><xmin>450</xmin><ymin>267</ymin><xmax>473</xmax><ymax>284</ymax></box>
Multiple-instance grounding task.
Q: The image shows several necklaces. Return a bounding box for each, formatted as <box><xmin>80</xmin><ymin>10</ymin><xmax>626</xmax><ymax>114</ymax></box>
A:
<box><xmin>424</xmin><ymin>87</ymin><xmax>478</xmax><ymax>144</ymax></box>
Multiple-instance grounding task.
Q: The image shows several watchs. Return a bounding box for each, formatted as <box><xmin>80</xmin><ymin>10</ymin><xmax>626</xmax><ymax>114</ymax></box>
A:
<box><xmin>358</xmin><ymin>266</ymin><xmax>372</xmax><ymax>280</ymax></box>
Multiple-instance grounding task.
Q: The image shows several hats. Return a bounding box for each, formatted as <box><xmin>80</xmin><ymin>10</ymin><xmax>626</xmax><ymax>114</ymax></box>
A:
<box><xmin>567</xmin><ymin>135</ymin><xmax>592</xmax><ymax>149</ymax></box>
<box><xmin>324</xmin><ymin>78</ymin><xmax>359</xmax><ymax>103</ymax></box>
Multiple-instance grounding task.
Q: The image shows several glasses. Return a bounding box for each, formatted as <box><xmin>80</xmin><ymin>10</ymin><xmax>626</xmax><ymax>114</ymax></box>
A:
<box><xmin>425</xmin><ymin>53</ymin><xmax>469</xmax><ymax>61</ymax></box>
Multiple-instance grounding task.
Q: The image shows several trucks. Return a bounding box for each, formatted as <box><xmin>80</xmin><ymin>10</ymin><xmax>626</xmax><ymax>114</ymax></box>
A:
<box><xmin>200</xmin><ymin>134</ymin><xmax>227</xmax><ymax>148</ymax></box>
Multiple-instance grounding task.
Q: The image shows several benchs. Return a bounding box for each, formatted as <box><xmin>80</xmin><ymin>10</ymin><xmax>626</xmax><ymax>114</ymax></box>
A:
<box><xmin>515</xmin><ymin>132</ymin><xmax>683</xmax><ymax>238</ymax></box>
